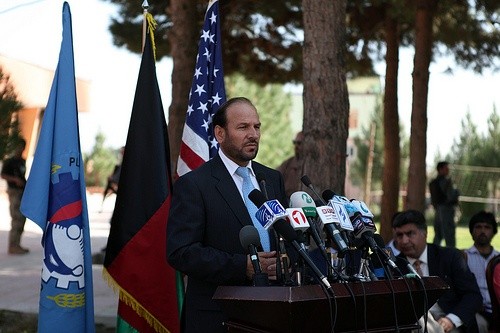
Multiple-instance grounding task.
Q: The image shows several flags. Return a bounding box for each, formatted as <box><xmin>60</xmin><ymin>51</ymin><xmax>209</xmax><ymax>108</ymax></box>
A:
<box><xmin>175</xmin><ymin>0</ymin><xmax>227</xmax><ymax>181</ymax></box>
<box><xmin>19</xmin><ymin>0</ymin><xmax>96</xmax><ymax>333</ymax></box>
<box><xmin>102</xmin><ymin>12</ymin><xmax>186</xmax><ymax>333</ymax></box>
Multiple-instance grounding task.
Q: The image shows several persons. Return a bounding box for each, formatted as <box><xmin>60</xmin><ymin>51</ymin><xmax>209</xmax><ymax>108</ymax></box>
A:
<box><xmin>0</xmin><ymin>137</ymin><xmax>30</xmax><ymax>254</ymax></box>
<box><xmin>387</xmin><ymin>161</ymin><xmax>500</xmax><ymax>333</ymax></box>
<box><xmin>165</xmin><ymin>97</ymin><xmax>289</xmax><ymax>333</ymax></box>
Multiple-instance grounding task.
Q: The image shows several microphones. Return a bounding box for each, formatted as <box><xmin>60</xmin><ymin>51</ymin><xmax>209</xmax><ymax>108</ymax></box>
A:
<box><xmin>240</xmin><ymin>176</ymin><xmax>423</xmax><ymax>296</ymax></box>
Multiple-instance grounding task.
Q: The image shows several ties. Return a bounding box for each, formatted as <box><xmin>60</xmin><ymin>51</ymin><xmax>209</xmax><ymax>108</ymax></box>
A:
<box><xmin>413</xmin><ymin>260</ymin><xmax>423</xmax><ymax>277</ymax></box>
<box><xmin>235</xmin><ymin>166</ymin><xmax>271</xmax><ymax>253</ymax></box>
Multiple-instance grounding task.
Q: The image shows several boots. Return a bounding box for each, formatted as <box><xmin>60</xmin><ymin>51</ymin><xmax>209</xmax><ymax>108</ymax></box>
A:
<box><xmin>8</xmin><ymin>230</ymin><xmax>31</xmax><ymax>256</ymax></box>
